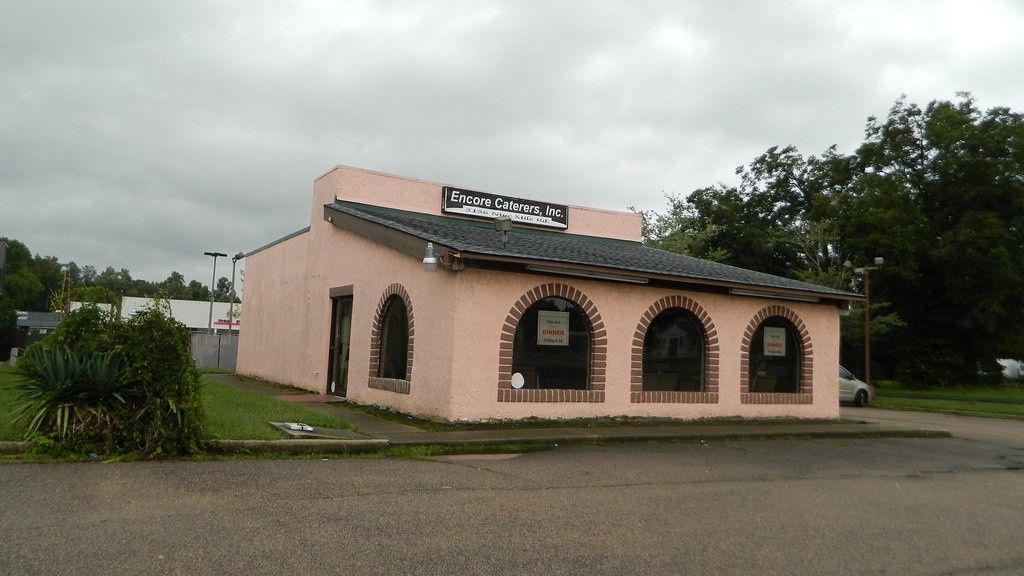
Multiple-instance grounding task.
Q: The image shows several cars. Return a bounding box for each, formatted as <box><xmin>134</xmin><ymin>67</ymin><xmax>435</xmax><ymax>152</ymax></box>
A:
<box><xmin>838</xmin><ymin>364</ymin><xmax>871</xmax><ymax>407</ymax></box>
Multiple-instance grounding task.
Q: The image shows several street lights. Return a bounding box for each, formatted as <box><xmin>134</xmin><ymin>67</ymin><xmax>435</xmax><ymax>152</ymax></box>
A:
<box><xmin>843</xmin><ymin>257</ymin><xmax>885</xmax><ymax>396</ymax></box>
<box><xmin>203</xmin><ymin>251</ymin><xmax>227</xmax><ymax>333</ymax></box>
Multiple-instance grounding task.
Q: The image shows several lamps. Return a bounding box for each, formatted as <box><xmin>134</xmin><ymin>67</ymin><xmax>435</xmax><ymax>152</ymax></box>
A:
<box><xmin>422</xmin><ymin>242</ymin><xmax>443</xmax><ymax>272</ymax></box>
<box><xmin>729</xmin><ymin>289</ymin><xmax>820</xmax><ymax>303</ymax></box>
<box><xmin>525</xmin><ymin>265</ymin><xmax>649</xmax><ymax>284</ymax></box>
